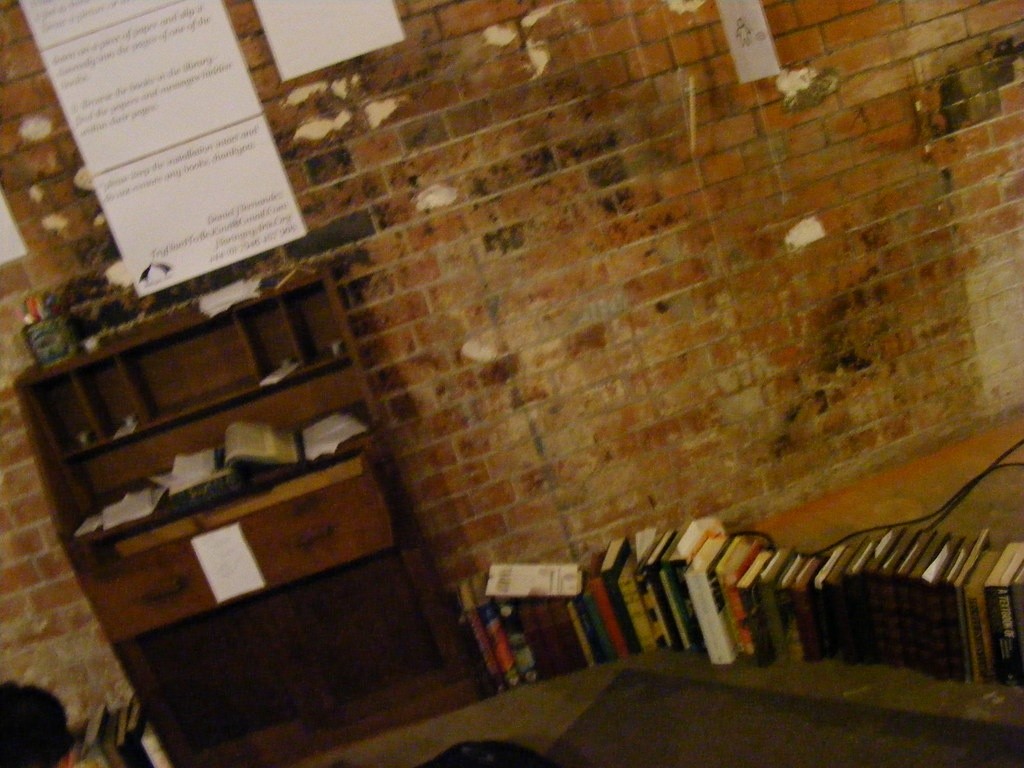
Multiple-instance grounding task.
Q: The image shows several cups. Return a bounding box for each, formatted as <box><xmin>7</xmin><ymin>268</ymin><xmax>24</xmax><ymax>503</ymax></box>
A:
<box><xmin>28</xmin><ymin>315</ymin><xmax>76</xmax><ymax>367</ymax></box>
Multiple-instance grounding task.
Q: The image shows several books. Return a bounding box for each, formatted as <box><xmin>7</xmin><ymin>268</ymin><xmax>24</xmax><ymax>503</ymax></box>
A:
<box><xmin>166</xmin><ymin>419</ymin><xmax>299</xmax><ymax>507</ymax></box>
<box><xmin>454</xmin><ymin>519</ymin><xmax>1024</xmax><ymax>700</ymax></box>
<box><xmin>64</xmin><ymin>696</ymin><xmax>173</xmax><ymax>768</ymax></box>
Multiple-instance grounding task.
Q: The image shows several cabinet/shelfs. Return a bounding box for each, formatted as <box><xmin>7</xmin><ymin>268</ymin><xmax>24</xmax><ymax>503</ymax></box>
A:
<box><xmin>10</xmin><ymin>259</ymin><xmax>493</xmax><ymax>768</ymax></box>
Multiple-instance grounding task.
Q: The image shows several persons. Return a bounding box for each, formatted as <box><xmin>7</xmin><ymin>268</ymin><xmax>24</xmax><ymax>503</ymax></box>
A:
<box><xmin>0</xmin><ymin>681</ymin><xmax>75</xmax><ymax>768</ymax></box>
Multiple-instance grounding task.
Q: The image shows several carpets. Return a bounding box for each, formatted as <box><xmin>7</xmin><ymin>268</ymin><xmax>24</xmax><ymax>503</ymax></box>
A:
<box><xmin>544</xmin><ymin>664</ymin><xmax>1024</xmax><ymax>768</ymax></box>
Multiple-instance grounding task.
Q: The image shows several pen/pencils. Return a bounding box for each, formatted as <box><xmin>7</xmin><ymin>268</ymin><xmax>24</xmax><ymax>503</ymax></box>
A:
<box><xmin>12</xmin><ymin>295</ymin><xmax>62</xmax><ymax>326</ymax></box>
